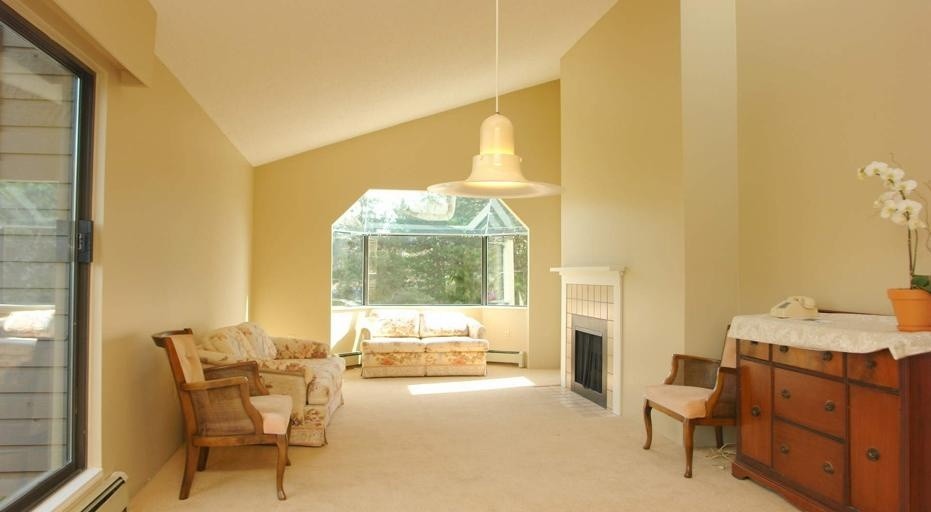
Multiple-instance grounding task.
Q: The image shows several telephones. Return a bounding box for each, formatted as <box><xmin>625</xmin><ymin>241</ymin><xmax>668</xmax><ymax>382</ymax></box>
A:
<box><xmin>770</xmin><ymin>295</ymin><xmax>818</xmax><ymax>320</ymax></box>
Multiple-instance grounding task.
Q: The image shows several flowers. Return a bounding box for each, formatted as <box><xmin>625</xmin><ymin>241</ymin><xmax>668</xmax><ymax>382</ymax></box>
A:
<box><xmin>857</xmin><ymin>151</ymin><xmax>931</xmax><ymax>296</ymax></box>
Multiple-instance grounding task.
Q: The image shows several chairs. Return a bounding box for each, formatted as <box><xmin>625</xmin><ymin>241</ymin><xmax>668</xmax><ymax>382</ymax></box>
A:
<box><xmin>642</xmin><ymin>353</ymin><xmax>737</xmax><ymax>478</ymax></box>
<box><xmin>151</xmin><ymin>328</ymin><xmax>294</xmax><ymax>500</ymax></box>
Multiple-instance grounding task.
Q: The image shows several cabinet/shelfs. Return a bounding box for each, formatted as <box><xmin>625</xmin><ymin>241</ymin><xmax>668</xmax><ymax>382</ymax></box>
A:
<box><xmin>727</xmin><ymin>310</ymin><xmax>931</xmax><ymax>512</ymax></box>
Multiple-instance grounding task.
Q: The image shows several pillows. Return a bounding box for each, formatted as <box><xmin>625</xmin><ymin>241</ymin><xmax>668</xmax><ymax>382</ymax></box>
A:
<box><xmin>369</xmin><ymin>310</ymin><xmax>420</xmax><ymax>338</ymax></box>
<box><xmin>237</xmin><ymin>323</ymin><xmax>278</xmax><ymax>360</ymax></box>
<box><xmin>201</xmin><ymin>324</ymin><xmax>256</xmax><ymax>360</ymax></box>
<box><xmin>420</xmin><ymin>312</ymin><xmax>469</xmax><ymax>338</ymax></box>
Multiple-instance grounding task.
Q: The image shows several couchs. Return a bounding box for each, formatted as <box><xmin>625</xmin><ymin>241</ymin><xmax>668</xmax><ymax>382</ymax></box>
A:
<box><xmin>359</xmin><ymin>309</ymin><xmax>489</xmax><ymax>378</ymax></box>
<box><xmin>196</xmin><ymin>335</ymin><xmax>346</xmax><ymax>448</ymax></box>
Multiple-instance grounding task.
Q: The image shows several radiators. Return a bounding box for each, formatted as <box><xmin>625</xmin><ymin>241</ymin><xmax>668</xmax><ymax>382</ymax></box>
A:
<box><xmin>70</xmin><ymin>471</ymin><xmax>128</xmax><ymax>512</ymax></box>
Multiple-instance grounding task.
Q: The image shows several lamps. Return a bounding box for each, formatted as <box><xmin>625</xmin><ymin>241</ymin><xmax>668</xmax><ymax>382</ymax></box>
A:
<box><xmin>426</xmin><ymin>0</ymin><xmax>567</xmax><ymax>198</ymax></box>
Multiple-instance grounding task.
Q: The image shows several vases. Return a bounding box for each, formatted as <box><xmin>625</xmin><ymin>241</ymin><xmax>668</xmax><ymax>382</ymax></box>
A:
<box><xmin>887</xmin><ymin>289</ymin><xmax>931</xmax><ymax>332</ymax></box>
